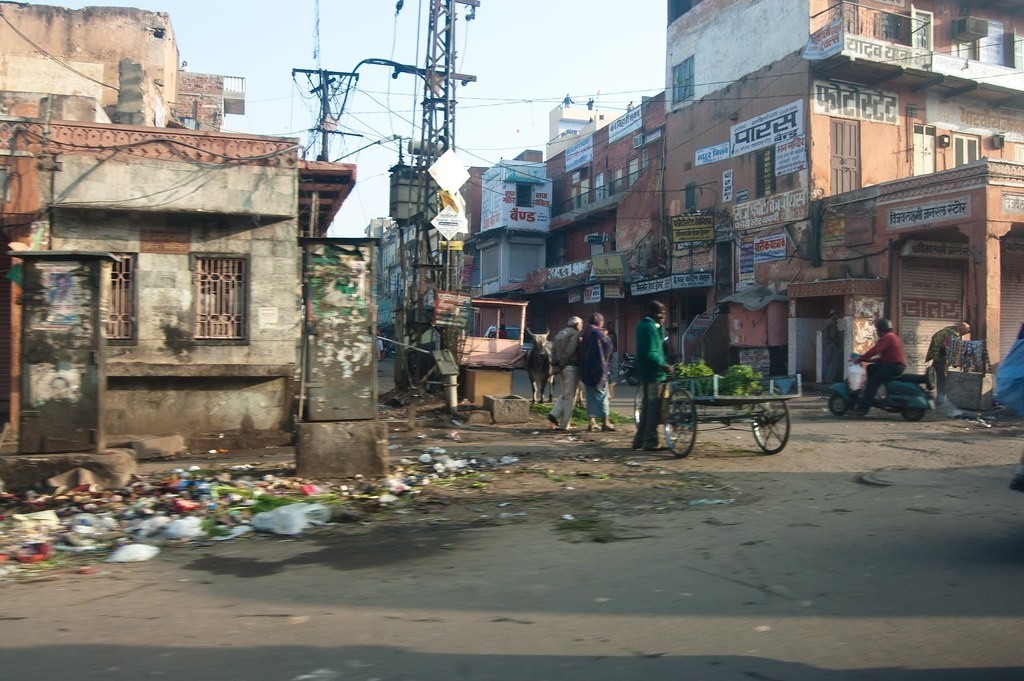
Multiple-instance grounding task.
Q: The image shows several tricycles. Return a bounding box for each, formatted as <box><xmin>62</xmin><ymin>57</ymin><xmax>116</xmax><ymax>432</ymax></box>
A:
<box><xmin>633</xmin><ymin>364</ymin><xmax>803</xmax><ymax>458</ymax></box>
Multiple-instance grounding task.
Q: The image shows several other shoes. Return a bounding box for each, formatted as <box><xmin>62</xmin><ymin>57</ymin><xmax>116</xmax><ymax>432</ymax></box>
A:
<box><xmin>560</xmin><ymin>427</ymin><xmax>577</xmax><ymax>432</ymax></box>
<box><xmin>589</xmin><ymin>424</ymin><xmax>602</xmax><ymax>432</ymax></box>
<box><xmin>848</xmin><ymin>409</ymin><xmax>867</xmax><ymax>415</ymax></box>
<box><xmin>547</xmin><ymin>414</ymin><xmax>558</xmax><ymax>426</ymax></box>
<box><xmin>602</xmin><ymin>424</ymin><xmax>615</xmax><ymax>431</ymax></box>
<box><xmin>633</xmin><ymin>444</ymin><xmax>645</xmax><ymax>451</ymax></box>
<box><xmin>644</xmin><ymin>444</ymin><xmax>671</xmax><ymax>451</ymax></box>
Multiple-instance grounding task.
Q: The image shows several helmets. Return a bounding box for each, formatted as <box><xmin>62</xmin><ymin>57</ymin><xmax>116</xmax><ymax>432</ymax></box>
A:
<box><xmin>876</xmin><ymin>319</ymin><xmax>893</xmax><ymax>332</ymax></box>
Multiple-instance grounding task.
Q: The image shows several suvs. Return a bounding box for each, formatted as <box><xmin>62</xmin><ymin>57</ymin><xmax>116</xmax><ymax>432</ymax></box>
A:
<box><xmin>484</xmin><ymin>324</ymin><xmax>535</xmax><ymax>353</ymax></box>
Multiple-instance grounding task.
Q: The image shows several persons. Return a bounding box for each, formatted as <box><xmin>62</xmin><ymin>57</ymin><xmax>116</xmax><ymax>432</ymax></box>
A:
<box><xmin>548</xmin><ymin>315</ymin><xmax>583</xmax><ymax>430</ymax></box>
<box><xmin>847</xmin><ymin>318</ymin><xmax>906</xmax><ymax>416</ymax></box>
<box><xmin>380</xmin><ymin>333</ymin><xmax>387</xmax><ymax>361</ymax></box>
<box><xmin>632</xmin><ymin>300</ymin><xmax>670</xmax><ymax>451</ymax></box>
<box><xmin>577</xmin><ymin>313</ymin><xmax>617</xmax><ymax>431</ymax></box>
<box><xmin>996</xmin><ymin>324</ymin><xmax>1024</xmax><ymax>492</ymax></box>
<box><xmin>926</xmin><ymin>322</ymin><xmax>969</xmax><ymax>406</ymax></box>
<box><xmin>494</xmin><ymin>324</ymin><xmax>508</xmax><ymax>339</ymax></box>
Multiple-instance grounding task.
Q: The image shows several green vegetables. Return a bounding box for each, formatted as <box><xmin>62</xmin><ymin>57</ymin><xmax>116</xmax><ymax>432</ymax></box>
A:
<box><xmin>528</xmin><ymin>401</ymin><xmax>636</xmax><ymax>426</ymax></box>
<box><xmin>671</xmin><ymin>357</ymin><xmax>764</xmax><ymax>396</ymax></box>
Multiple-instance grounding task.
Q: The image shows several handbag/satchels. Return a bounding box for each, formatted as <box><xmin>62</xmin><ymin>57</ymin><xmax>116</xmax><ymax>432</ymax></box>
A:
<box><xmin>847</xmin><ymin>361</ymin><xmax>867</xmax><ymax>391</ymax></box>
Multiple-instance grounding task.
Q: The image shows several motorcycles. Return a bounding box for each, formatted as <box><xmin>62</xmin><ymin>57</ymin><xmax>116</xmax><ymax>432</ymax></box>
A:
<box><xmin>617</xmin><ymin>351</ymin><xmax>640</xmax><ymax>385</ymax></box>
<box><xmin>828</xmin><ymin>353</ymin><xmax>933</xmax><ymax>422</ymax></box>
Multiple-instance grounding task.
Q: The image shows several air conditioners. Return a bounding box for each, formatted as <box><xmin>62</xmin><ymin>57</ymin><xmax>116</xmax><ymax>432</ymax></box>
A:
<box><xmin>633</xmin><ymin>134</ymin><xmax>648</xmax><ymax>149</ymax></box>
<box><xmin>956</xmin><ymin>16</ymin><xmax>988</xmax><ymax>41</ymax></box>
